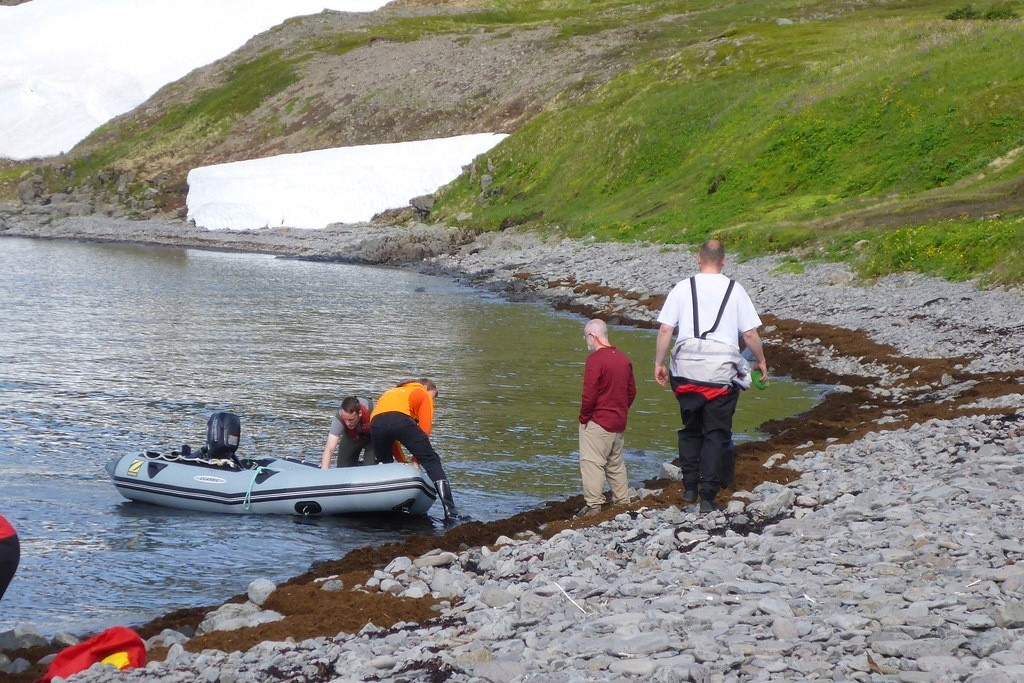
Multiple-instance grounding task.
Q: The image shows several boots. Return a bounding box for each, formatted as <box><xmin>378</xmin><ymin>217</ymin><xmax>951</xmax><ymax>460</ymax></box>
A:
<box><xmin>432</xmin><ymin>480</ymin><xmax>457</xmax><ymax>520</ymax></box>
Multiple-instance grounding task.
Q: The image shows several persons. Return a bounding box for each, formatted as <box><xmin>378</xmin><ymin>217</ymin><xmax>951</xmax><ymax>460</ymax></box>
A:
<box><xmin>321</xmin><ymin>397</ymin><xmax>378</xmax><ymax>468</ymax></box>
<box><xmin>655</xmin><ymin>239</ymin><xmax>768</xmax><ymax>513</ymax></box>
<box><xmin>370</xmin><ymin>379</ymin><xmax>460</xmax><ymax>517</ymax></box>
<box><xmin>578</xmin><ymin>319</ymin><xmax>637</xmax><ymax>517</ymax></box>
<box><xmin>0</xmin><ymin>515</ymin><xmax>20</xmax><ymax>600</ymax></box>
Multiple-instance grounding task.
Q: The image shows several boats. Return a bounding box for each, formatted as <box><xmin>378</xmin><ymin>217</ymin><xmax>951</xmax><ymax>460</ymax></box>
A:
<box><xmin>105</xmin><ymin>453</ymin><xmax>438</xmax><ymax>525</ymax></box>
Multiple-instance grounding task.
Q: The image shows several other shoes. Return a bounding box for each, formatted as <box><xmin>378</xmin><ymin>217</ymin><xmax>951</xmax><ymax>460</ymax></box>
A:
<box><xmin>682</xmin><ymin>487</ymin><xmax>698</xmax><ymax>502</ymax></box>
<box><xmin>699</xmin><ymin>499</ymin><xmax>716</xmax><ymax>512</ymax></box>
<box><xmin>582</xmin><ymin>506</ymin><xmax>601</xmax><ymax>517</ymax></box>
